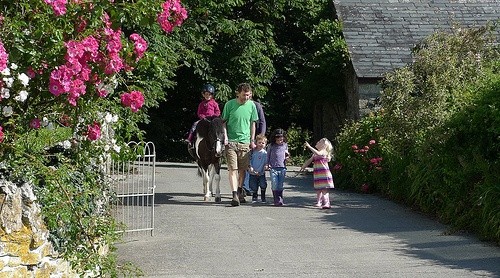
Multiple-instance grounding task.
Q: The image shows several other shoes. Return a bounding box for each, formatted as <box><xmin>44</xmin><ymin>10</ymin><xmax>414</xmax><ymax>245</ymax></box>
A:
<box><xmin>231</xmin><ymin>192</ymin><xmax>240</xmax><ymax>206</ymax></box>
<box><xmin>252</xmin><ymin>195</ymin><xmax>258</xmax><ymax>203</ymax></box>
<box><xmin>315</xmin><ymin>200</ymin><xmax>323</xmax><ymax>207</ymax></box>
<box><xmin>185</xmin><ymin>139</ymin><xmax>190</xmax><ymax>142</ymax></box>
<box><xmin>274</xmin><ymin>196</ymin><xmax>285</xmax><ymax>206</ymax></box>
<box><xmin>261</xmin><ymin>196</ymin><xmax>267</xmax><ymax>204</ymax></box>
<box><xmin>323</xmin><ymin>200</ymin><xmax>332</xmax><ymax>209</ymax></box>
<box><xmin>237</xmin><ymin>188</ymin><xmax>246</xmax><ymax>203</ymax></box>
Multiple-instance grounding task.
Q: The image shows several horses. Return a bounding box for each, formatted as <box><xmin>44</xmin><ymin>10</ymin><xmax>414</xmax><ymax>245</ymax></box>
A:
<box><xmin>188</xmin><ymin>115</ymin><xmax>228</xmax><ymax>203</ymax></box>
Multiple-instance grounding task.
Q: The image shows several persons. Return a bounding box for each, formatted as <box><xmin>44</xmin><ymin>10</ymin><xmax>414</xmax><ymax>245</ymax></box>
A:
<box><xmin>267</xmin><ymin>129</ymin><xmax>290</xmax><ymax>207</ymax></box>
<box><xmin>302</xmin><ymin>138</ymin><xmax>334</xmax><ymax>209</ymax></box>
<box><xmin>185</xmin><ymin>85</ymin><xmax>221</xmax><ymax>146</ymax></box>
<box><xmin>222</xmin><ymin>82</ymin><xmax>267</xmax><ymax>206</ymax></box>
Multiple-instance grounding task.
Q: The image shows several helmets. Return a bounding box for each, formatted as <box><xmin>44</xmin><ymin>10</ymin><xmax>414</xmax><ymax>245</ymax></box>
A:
<box><xmin>203</xmin><ymin>84</ymin><xmax>215</xmax><ymax>93</ymax></box>
